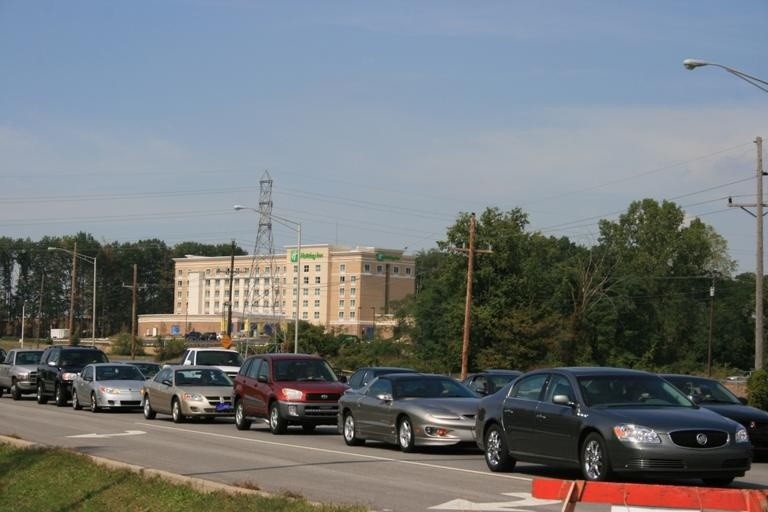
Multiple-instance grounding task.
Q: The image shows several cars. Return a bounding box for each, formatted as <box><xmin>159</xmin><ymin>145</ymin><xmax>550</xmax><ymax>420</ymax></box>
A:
<box><xmin>186</xmin><ymin>331</ymin><xmax>216</xmax><ymax>341</ymax></box>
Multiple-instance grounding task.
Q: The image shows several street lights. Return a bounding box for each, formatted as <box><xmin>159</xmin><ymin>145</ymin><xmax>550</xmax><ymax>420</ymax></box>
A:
<box><xmin>47</xmin><ymin>246</ymin><xmax>97</xmax><ymax>347</ymax></box>
<box><xmin>21</xmin><ymin>300</ymin><xmax>28</xmax><ymax>348</ymax></box>
<box><xmin>682</xmin><ymin>57</ymin><xmax>768</xmax><ymax>95</ymax></box>
<box><xmin>233</xmin><ymin>204</ymin><xmax>302</xmax><ymax>354</ymax></box>
